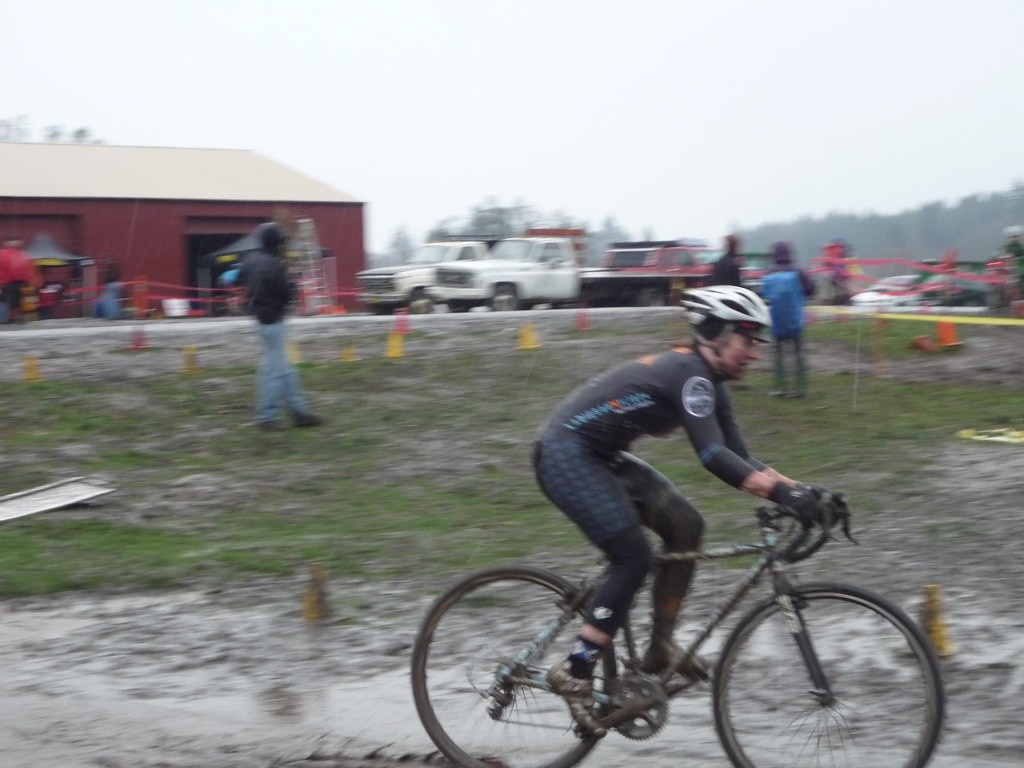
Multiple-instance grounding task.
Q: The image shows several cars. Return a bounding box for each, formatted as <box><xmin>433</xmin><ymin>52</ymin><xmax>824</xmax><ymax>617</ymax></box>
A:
<box><xmin>913</xmin><ymin>260</ymin><xmax>1004</xmax><ymax>309</ymax></box>
<box><xmin>603</xmin><ymin>244</ymin><xmax>776</xmax><ymax>307</ymax></box>
<box><xmin>851</xmin><ymin>276</ymin><xmax>924</xmax><ymax>308</ymax></box>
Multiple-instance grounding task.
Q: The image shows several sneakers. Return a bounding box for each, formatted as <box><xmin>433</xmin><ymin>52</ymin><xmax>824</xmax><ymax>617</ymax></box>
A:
<box><xmin>547</xmin><ymin>662</ymin><xmax>608</xmax><ymax>739</ymax></box>
<box><xmin>640</xmin><ymin>642</ymin><xmax>710</xmax><ymax>677</ymax></box>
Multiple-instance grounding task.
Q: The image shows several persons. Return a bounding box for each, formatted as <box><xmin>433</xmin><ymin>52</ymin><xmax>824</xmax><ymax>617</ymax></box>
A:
<box><xmin>234</xmin><ymin>223</ymin><xmax>328</xmax><ymax>430</ymax></box>
<box><xmin>0</xmin><ymin>236</ymin><xmax>33</xmax><ymax>325</ymax></box>
<box><xmin>759</xmin><ymin>239</ymin><xmax>821</xmax><ymax>398</ymax></box>
<box><xmin>712</xmin><ymin>235</ymin><xmax>742</xmax><ymax>286</ymax></box>
<box><xmin>532</xmin><ymin>284</ymin><xmax>848</xmax><ymax>738</ymax></box>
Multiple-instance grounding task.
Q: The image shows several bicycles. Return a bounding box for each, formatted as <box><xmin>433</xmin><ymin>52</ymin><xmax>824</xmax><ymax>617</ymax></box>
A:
<box><xmin>410</xmin><ymin>487</ymin><xmax>946</xmax><ymax>768</ymax></box>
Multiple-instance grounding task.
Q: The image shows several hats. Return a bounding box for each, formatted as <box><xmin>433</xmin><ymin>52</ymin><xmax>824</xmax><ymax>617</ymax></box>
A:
<box><xmin>262</xmin><ymin>228</ymin><xmax>283</xmax><ymax>246</ymax></box>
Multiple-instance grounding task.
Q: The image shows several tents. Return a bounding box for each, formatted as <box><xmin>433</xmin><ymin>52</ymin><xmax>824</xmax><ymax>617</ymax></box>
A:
<box><xmin>197</xmin><ymin>223</ymin><xmax>330</xmax><ymax>268</ymax></box>
<box><xmin>23</xmin><ymin>231</ymin><xmax>97</xmax><ymax>266</ymax></box>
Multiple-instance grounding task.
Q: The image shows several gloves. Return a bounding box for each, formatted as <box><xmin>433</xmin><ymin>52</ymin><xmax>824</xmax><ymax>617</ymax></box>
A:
<box><xmin>770</xmin><ymin>480</ymin><xmax>850</xmax><ymax>531</ymax></box>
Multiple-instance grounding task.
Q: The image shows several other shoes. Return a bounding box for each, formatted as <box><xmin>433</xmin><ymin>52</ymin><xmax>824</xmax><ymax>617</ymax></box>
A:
<box><xmin>259</xmin><ymin>414</ymin><xmax>328</xmax><ymax>433</ymax></box>
<box><xmin>769</xmin><ymin>384</ymin><xmax>806</xmax><ymax>398</ymax></box>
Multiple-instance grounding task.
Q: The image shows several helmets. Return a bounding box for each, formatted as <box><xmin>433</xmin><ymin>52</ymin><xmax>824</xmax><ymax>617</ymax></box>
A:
<box><xmin>679</xmin><ymin>284</ymin><xmax>774</xmax><ymax>349</ymax></box>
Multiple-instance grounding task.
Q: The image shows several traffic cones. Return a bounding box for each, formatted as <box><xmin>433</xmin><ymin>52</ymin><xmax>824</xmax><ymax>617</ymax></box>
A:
<box><xmin>301</xmin><ymin>566</ymin><xmax>332</xmax><ymax>618</ymax></box>
<box><xmin>902</xmin><ymin>585</ymin><xmax>958</xmax><ymax>657</ymax></box>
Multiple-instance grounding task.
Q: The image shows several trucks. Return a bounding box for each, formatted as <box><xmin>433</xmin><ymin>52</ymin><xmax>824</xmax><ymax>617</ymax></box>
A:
<box><xmin>357</xmin><ymin>240</ymin><xmax>489</xmax><ymax>315</ymax></box>
<box><xmin>425</xmin><ymin>237</ymin><xmax>711</xmax><ymax>313</ymax></box>
